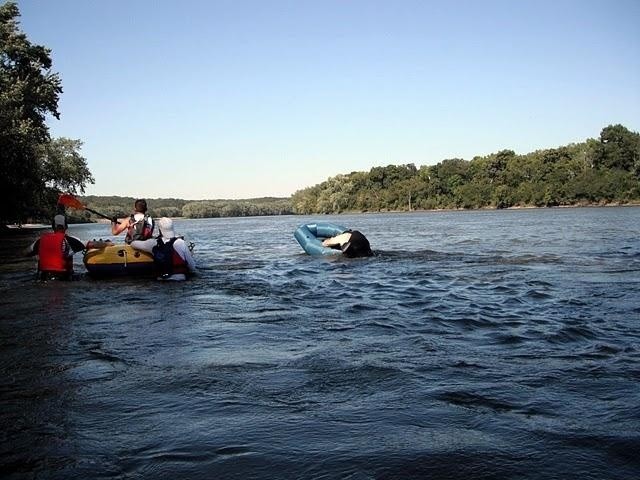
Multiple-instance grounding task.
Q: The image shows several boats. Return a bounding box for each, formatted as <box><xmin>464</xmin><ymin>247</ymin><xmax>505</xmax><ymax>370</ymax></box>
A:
<box><xmin>83</xmin><ymin>240</ymin><xmax>160</xmax><ymax>279</ymax></box>
<box><xmin>6</xmin><ymin>223</ymin><xmax>53</xmax><ymax>229</ymax></box>
<box><xmin>294</xmin><ymin>221</ymin><xmax>352</xmax><ymax>260</ymax></box>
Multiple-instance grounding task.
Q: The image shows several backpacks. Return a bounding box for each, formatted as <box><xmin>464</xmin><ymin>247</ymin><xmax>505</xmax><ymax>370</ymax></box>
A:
<box><xmin>129</xmin><ymin>214</ymin><xmax>148</xmax><ymax>241</ymax></box>
<box><xmin>153</xmin><ymin>238</ymin><xmax>173</xmax><ymax>279</ymax></box>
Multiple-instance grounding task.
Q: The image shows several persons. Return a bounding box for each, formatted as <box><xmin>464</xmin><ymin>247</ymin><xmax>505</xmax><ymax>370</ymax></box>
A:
<box><xmin>323</xmin><ymin>229</ymin><xmax>371</xmax><ymax>258</ymax></box>
<box><xmin>111</xmin><ymin>199</ymin><xmax>195</xmax><ymax>281</ymax></box>
<box><xmin>22</xmin><ymin>215</ymin><xmax>87</xmax><ymax>282</ymax></box>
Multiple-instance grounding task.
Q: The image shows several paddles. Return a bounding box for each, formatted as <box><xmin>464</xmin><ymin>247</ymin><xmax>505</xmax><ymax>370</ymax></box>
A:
<box><xmin>57</xmin><ymin>193</ymin><xmax>121</xmax><ymax>224</ymax></box>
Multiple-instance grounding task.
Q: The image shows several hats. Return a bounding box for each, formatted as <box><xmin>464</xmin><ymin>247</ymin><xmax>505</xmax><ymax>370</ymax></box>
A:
<box><xmin>158</xmin><ymin>217</ymin><xmax>175</xmax><ymax>239</ymax></box>
<box><xmin>51</xmin><ymin>214</ymin><xmax>69</xmax><ymax>230</ymax></box>
